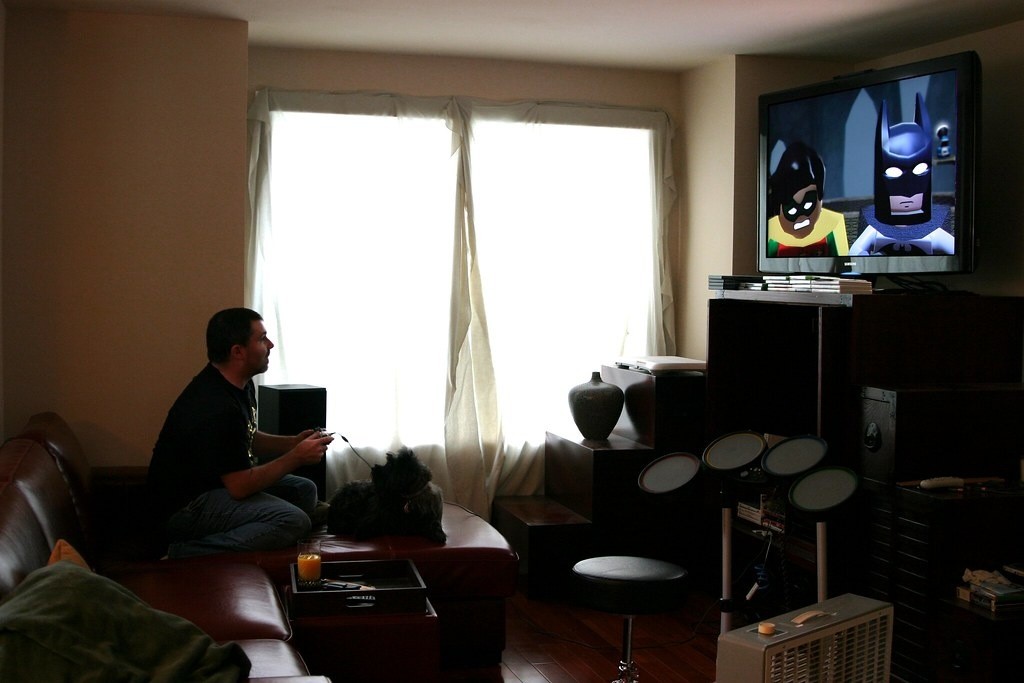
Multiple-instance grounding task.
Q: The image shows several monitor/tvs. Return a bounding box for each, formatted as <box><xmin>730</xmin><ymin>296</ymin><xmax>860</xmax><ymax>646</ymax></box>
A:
<box><xmin>759</xmin><ymin>48</ymin><xmax>985</xmax><ymax>276</ymax></box>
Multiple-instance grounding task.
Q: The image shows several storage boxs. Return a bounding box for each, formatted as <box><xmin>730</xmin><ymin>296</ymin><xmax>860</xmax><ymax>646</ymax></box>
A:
<box><xmin>289</xmin><ymin>559</ymin><xmax>427</xmax><ymax>615</ymax></box>
<box><xmin>861</xmin><ymin>385</ymin><xmax>1024</xmax><ymax>478</ymax></box>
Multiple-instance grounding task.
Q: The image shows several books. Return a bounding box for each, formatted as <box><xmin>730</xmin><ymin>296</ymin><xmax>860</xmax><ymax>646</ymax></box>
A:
<box><xmin>956</xmin><ymin>562</ymin><xmax>1024</xmax><ymax>612</ymax></box>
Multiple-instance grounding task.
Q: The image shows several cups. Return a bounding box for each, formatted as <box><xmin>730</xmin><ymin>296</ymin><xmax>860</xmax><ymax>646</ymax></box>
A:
<box><xmin>297</xmin><ymin>538</ymin><xmax>320</xmax><ymax>585</ymax></box>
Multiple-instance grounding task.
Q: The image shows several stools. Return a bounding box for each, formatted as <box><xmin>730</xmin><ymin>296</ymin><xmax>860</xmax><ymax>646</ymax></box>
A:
<box><xmin>571</xmin><ymin>556</ymin><xmax>684</xmax><ymax>683</ymax></box>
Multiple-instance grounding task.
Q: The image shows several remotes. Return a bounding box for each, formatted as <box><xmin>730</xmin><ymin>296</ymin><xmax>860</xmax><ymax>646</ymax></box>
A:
<box><xmin>920</xmin><ymin>476</ymin><xmax>964</xmax><ymax>488</ymax></box>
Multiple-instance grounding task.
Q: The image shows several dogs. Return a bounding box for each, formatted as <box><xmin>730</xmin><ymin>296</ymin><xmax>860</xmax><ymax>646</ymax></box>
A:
<box><xmin>327</xmin><ymin>452</ymin><xmax>446</xmax><ymax>545</ymax></box>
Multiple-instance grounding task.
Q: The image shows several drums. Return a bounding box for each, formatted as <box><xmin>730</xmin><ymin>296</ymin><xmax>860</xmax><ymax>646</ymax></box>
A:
<box><xmin>759</xmin><ymin>432</ymin><xmax>829</xmax><ymax>479</ymax></box>
<box><xmin>787</xmin><ymin>463</ymin><xmax>860</xmax><ymax>514</ymax></box>
<box><xmin>701</xmin><ymin>430</ymin><xmax>768</xmax><ymax>474</ymax></box>
<box><xmin>635</xmin><ymin>451</ymin><xmax>701</xmax><ymax>494</ymax></box>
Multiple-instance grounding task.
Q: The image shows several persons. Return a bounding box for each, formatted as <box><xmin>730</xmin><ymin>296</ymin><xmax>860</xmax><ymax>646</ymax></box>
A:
<box><xmin>138</xmin><ymin>307</ymin><xmax>334</xmax><ymax>562</ymax></box>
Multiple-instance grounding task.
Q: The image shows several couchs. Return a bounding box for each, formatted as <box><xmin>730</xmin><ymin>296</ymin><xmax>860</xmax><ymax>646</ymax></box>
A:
<box><xmin>18</xmin><ymin>411</ymin><xmax>519</xmax><ymax>668</ymax></box>
<box><xmin>0</xmin><ymin>437</ymin><xmax>310</xmax><ymax>683</ymax></box>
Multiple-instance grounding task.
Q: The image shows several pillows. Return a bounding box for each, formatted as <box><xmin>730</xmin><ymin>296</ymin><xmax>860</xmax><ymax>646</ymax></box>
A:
<box><xmin>48</xmin><ymin>538</ymin><xmax>91</xmax><ymax>570</ymax></box>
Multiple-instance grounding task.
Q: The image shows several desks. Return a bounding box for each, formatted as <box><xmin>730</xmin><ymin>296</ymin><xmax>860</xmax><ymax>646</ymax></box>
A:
<box><xmin>284</xmin><ymin>585</ymin><xmax>438</xmax><ymax>683</ymax></box>
